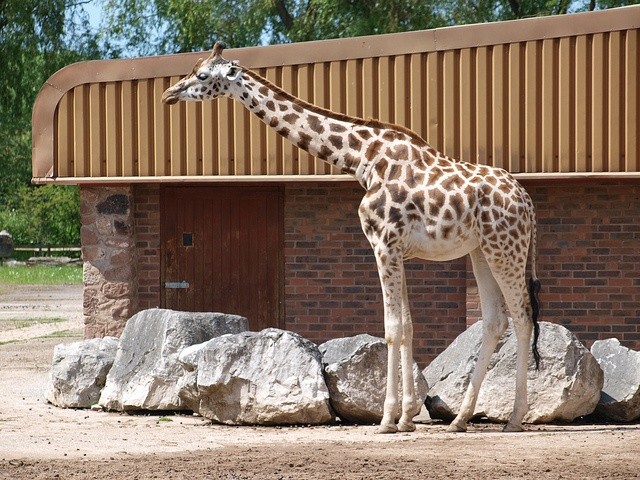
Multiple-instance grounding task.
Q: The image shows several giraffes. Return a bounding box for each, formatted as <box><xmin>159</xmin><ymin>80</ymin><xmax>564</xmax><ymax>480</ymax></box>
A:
<box><xmin>164</xmin><ymin>40</ymin><xmax>541</xmax><ymax>434</ymax></box>
